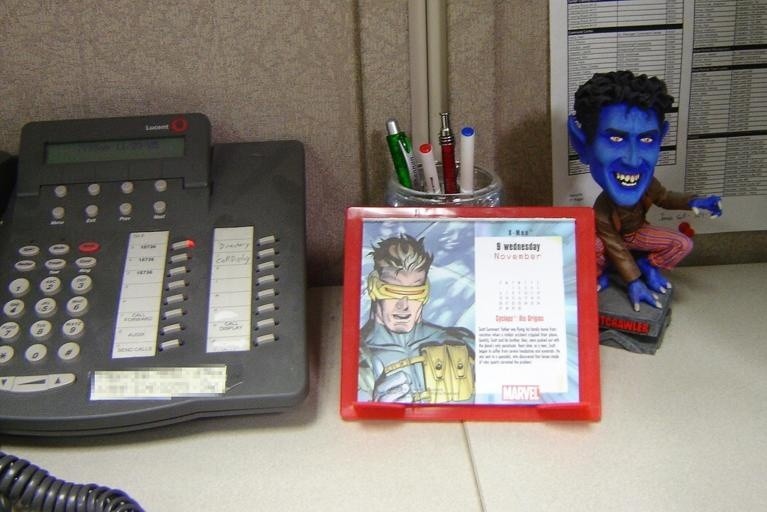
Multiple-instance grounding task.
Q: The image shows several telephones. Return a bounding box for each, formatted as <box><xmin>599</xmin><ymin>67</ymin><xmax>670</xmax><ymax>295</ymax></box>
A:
<box><xmin>0</xmin><ymin>112</ymin><xmax>309</xmax><ymax>436</ymax></box>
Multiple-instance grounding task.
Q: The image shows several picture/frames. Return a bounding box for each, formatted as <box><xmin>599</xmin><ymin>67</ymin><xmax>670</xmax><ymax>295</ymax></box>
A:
<box><xmin>337</xmin><ymin>204</ymin><xmax>603</xmax><ymax>424</ymax></box>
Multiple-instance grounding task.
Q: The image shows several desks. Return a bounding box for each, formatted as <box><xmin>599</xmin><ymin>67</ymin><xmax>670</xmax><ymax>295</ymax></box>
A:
<box><xmin>0</xmin><ymin>243</ymin><xmax>482</xmax><ymax>511</ymax></box>
<box><xmin>446</xmin><ymin>232</ymin><xmax>766</xmax><ymax>510</ymax></box>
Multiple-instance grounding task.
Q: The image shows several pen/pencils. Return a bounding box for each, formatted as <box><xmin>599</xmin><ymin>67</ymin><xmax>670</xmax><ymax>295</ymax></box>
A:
<box><xmin>385</xmin><ymin>113</ymin><xmax>475</xmax><ymax>194</ymax></box>
